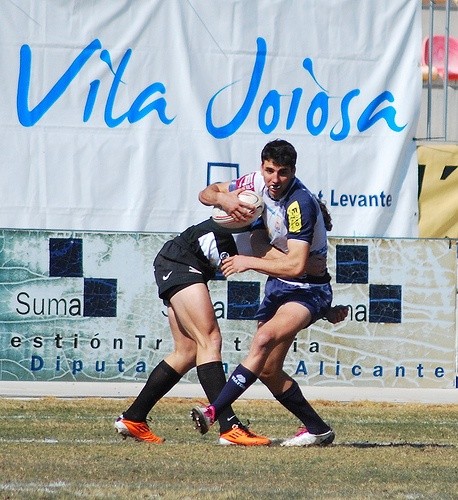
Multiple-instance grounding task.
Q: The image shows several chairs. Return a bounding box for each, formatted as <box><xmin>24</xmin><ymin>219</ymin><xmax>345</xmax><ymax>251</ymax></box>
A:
<box><xmin>422</xmin><ymin>34</ymin><xmax>458</xmax><ymax>80</ymax></box>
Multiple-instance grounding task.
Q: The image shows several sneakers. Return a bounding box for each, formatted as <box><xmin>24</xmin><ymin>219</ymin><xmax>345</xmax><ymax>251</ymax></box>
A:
<box><xmin>190</xmin><ymin>404</ymin><xmax>216</xmax><ymax>434</ymax></box>
<box><xmin>112</xmin><ymin>412</ymin><xmax>166</xmax><ymax>444</ymax></box>
<box><xmin>279</xmin><ymin>426</ymin><xmax>335</xmax><ymax>447</ymax></box>
<box><xmin>218</xmin><ymin>423</ymin><xmax>271</xmax><ymax>447</ymax></box>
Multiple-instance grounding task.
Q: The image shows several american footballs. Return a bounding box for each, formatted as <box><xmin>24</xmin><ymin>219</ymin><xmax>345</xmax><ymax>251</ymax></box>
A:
<box><xmin>211</xmin><ymin>190</ymin><xmax>264</xmax><ymax>229</ymax></box>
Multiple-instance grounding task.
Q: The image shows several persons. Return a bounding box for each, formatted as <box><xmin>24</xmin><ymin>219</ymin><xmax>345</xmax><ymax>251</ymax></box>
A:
<box><xmin>114</xmin><ymin>184</ymin><xmax>335</xmax><ymax>449</ymax></box>
<box><xmin>190</xmin><ymin>140</ymin><xmax>336</xmax><ymax>449</ymax></box>
